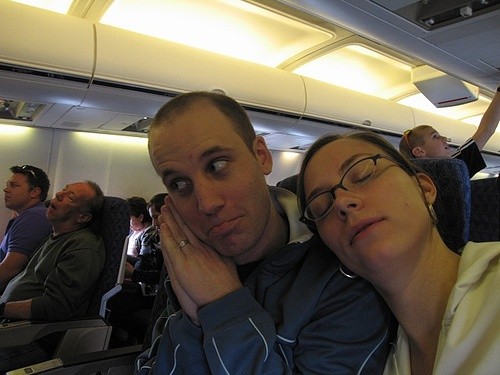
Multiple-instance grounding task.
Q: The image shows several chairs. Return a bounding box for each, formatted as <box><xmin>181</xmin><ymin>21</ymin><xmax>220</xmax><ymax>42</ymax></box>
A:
<box><xmin>1</xmin><ymin>158</ymin><xmax>470</xmax><ymax>375</ymax></box>
<box><xmin>470</xmin><ymin>178</ymin><xmax>500</xmax><ymax>243</ymax></box>
<box><xmin>0</xmin><ymin>196</ymin><xmax>133</xmax><ymax>360</ymax></box>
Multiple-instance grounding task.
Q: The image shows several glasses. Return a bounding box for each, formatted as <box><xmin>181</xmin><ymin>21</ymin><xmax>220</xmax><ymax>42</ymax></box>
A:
<box><xmin>298</xmin><ymin>153</ymin><xmax>410</xmax><ymax>231</ymax></box>
<box><xmin>403</xmin><ymin>129</ymin><xmax>412</xmax><ymax>149</ymax></box>
<box><xmin>14</xmin><ymin>165</ymin><xmax>37</xmax><ymax>181</ymax></box>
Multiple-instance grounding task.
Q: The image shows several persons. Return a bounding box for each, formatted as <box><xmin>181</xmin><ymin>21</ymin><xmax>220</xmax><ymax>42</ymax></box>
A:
<box><xmin>123</xmin><ymin>195</ymin><xmax>152</xmax><ymax>281</ymax></box>
<box><xmin>134</xmin><ymin>87</ymin><xmax>398</xmax><ymax>375</ymax></box>
<box><xmin>293</xmin><ymin>126</ymin><xmax>500</xmax><ymax>373</ymax></box>
<box><xmin>147</xmin><ymin>191</ymin><xmax>171</xmax><ymax>259</ymax></box>
<box><xmin>390</xmin><ymin>87</ymin><xmax>500</xmax><ymax>180</ymax></box>
<box><xmin>0</xmin><ymin>180</ymin><xmax>105</xmax><ymax>370</ymax></box>
<box><xmin>0</xmin><ymin>164</ymin><xmax>52</xmax><ymax>295</ymax></box>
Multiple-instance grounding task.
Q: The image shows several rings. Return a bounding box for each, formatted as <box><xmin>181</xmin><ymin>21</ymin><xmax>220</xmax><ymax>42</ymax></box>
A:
<box><xmin>180</xmin><ymin>239</ymin><xmax>188</xmax><ymax>246</ymax></box>
